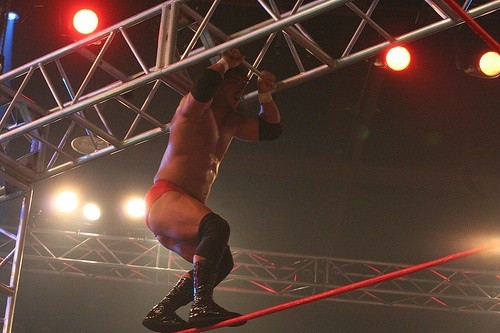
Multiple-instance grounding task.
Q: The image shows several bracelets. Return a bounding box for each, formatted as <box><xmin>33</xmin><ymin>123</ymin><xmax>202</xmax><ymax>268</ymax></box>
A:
<box><xmin>218</xmin><ymin>58</ymin><xmax>229</xmax><ymax>73</ymax></box>
<box><xmin>259</xmin><ymin>93</ymin><xmax>273</xmax><ymax>104</ymax></box>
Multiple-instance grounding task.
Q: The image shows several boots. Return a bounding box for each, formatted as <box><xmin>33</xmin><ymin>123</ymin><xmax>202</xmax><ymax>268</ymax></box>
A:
<box><xmin>142</xmin><ymin>277</ymin><xmax>195</xmax><ymax>332</ymax></box>
<box><xmin>187</xmin><ymin>258</ymin><xmax>246</xmax><ymax>327</ymax></box>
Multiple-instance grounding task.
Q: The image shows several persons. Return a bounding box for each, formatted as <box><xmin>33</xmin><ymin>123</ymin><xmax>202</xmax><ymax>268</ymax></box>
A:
<box><xmin>142</xmin><ymin>49</ymin><xmax>283</xmax><ymax>333</ymax></box>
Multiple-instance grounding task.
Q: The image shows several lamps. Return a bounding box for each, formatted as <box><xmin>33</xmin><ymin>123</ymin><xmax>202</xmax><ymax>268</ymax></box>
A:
<box><xmin>455</xmin><ymin>47</ymin><xmax>500</xmax><ymax>79</ymax></box>
